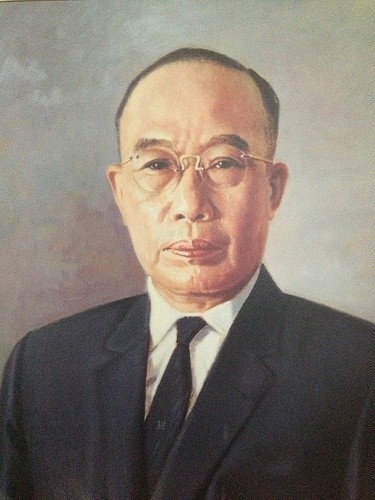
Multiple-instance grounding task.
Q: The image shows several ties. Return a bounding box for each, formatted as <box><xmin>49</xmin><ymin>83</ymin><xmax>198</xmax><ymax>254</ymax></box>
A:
<box><xmin>144</xmin><ymin>317</ymin><xmax>207</xmax><ymax>500</ymax></box>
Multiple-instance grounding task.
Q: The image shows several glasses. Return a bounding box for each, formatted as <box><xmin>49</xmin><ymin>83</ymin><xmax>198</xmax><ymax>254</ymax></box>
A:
<box><xmin>119</xmin><ymin>144</ymin><xmax>275</xmax><ymax>190</ymax></box>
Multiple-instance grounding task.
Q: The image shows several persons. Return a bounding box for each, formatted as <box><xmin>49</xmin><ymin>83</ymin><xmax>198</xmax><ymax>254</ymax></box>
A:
<box><xmin>0</xmin><ymin>46</ymin><xmax>375</xmax><ymax>500</ymax></box>
